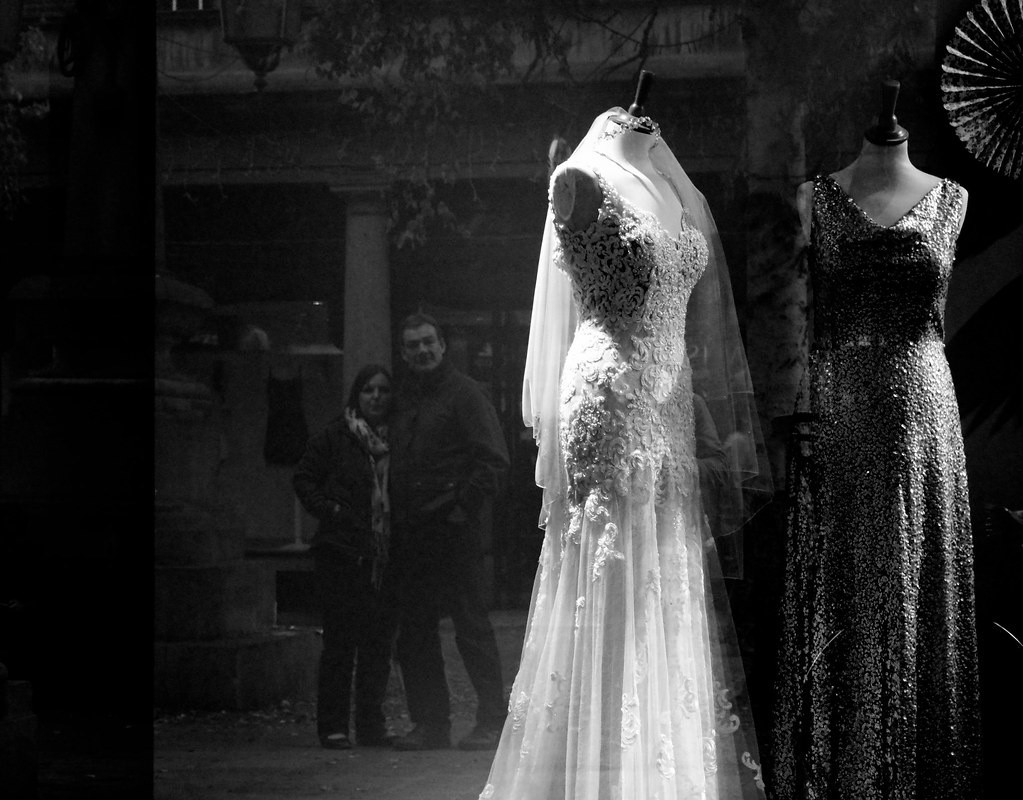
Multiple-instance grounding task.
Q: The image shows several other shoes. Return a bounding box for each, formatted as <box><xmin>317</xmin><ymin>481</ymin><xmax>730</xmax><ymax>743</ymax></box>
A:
<box><xmin>397</xmin><ymin>723</ymin><xmax>454</xmax><ymax>749</ymax></box>
<box><xmin>323</xmin><ymin>734</ymin><xmax>351</xmax><ymax>748</ymax></box>
<box><xmin>357</xmin><ymin>726</ymin><xmax>399</xmax><ymax>742</ymax></box>
<box><xmin>458</xmin><ymin>721</ymin><xmax>502</xmax><ymax>750</ymax></box>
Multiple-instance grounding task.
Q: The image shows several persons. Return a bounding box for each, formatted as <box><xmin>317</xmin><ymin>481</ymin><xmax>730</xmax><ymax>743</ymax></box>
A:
<box><xmin>387</xmin><ymin>312</ymin><xmax>508</xmax><ymax>751</ymax></box>
<box><xmin>716</xmin><ymin>431</ymin><xmax>759</xmax><ymax>653</ymax></box>
<box><xmin>691</xmin><ymin>392</ymin><xmax>729</xmax><ymax>547</ymax></box>
<box><xmin>770</xmin><ymin>80</ymin><xmax>987</xmax><ymax>800</ymax></box>
<box><xmin>290</xmin><ymin>365</ymin><xmax>397</xmax><ymax>751</ymax></box>
<box><xmin>547</xmin><ymin>68</ymin><xmax>736</xmax><ymax>800</ymax></box>
